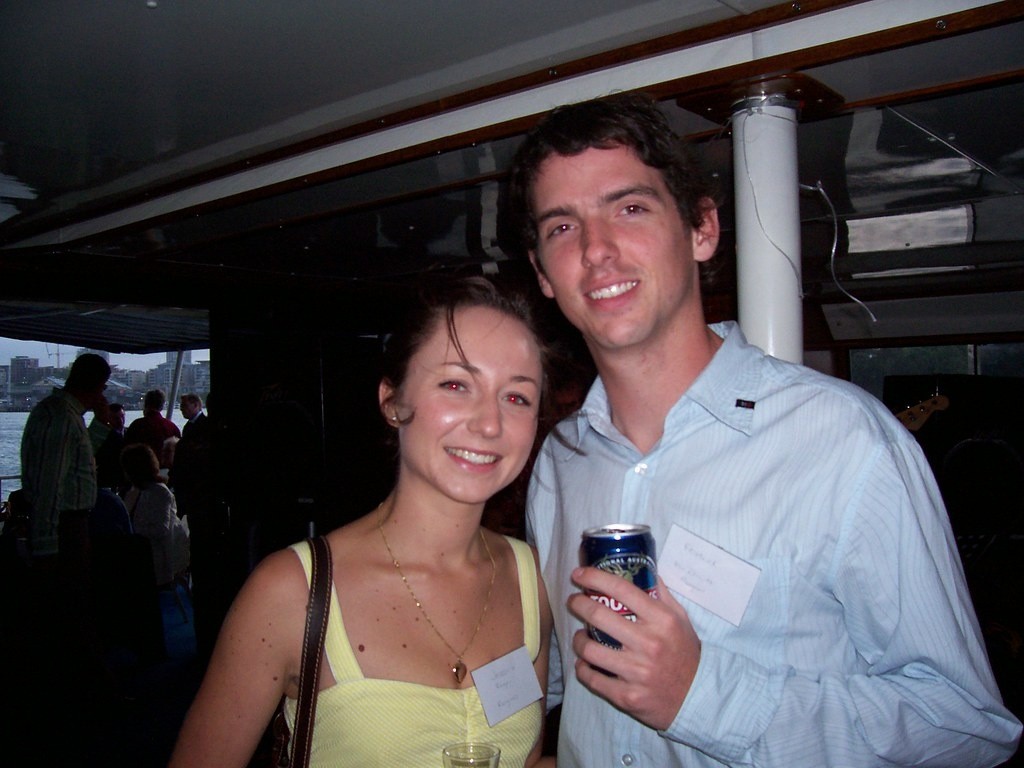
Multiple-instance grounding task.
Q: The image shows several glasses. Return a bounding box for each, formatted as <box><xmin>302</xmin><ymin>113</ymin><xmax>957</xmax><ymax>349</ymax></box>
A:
<box><xmin>94</xmin><ymin>385</ymin><xmax>107</xmax><ymax>390</ymax></box>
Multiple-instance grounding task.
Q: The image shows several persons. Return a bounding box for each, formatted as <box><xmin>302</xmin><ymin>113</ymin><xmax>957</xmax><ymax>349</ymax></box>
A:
<box><xmin>20</xmin><ymin>353</ymin><xmax>215</xmax><ymax>694</ymax></box>
<box><xmin>170</xmin><ymin>270</ymin><xmax>561</xmax><ymax>768</ymax></box>
<box><xmin>502</xmin><ymin>92</ymin><xmax>1024</xmax><ymax>768</ymax></box>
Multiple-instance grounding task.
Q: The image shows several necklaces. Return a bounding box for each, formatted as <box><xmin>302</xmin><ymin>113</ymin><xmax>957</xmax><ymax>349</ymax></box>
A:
<box><xmin>377</xmin><ymin>500</ymin><xmax>496</xmax><ymax>683</ymax></box>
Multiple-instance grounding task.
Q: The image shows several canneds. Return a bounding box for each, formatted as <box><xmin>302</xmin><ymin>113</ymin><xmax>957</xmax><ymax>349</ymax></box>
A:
<box><xmin>579</xmin><ymin>525</ymin><xmax>660</xmax><ymax>678</ymax></box>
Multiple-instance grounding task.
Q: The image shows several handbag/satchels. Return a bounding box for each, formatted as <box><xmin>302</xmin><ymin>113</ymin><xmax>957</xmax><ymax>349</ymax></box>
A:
<box><xmin>248</xmin><ymin>535</ymin><xmax>332</xmax><ymax>768</ymax></box>
<box><xmin>7</xmin><ymin>490</ymin><xmax>59</xmax><ymax>555</ymax></box>
<box><xmin>159</xmin><ymin>582</ymin><xmax>196</xmax><ymax>661</ymax></box>
